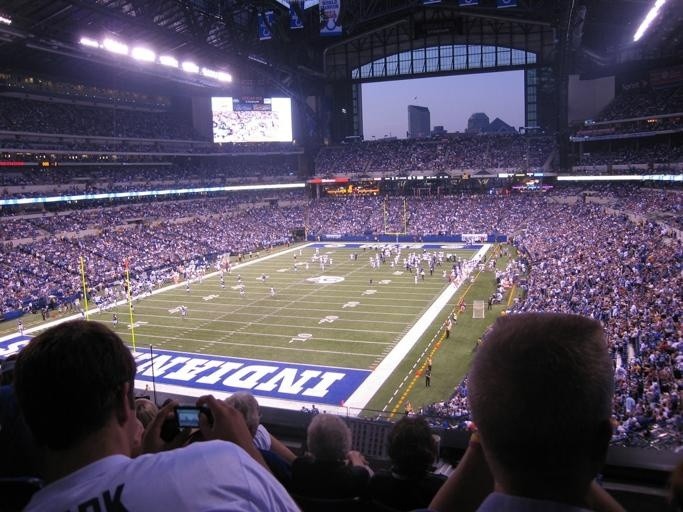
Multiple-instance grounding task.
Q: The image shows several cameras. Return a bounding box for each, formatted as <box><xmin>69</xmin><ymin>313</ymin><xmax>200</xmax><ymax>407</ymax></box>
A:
<box><xmin>174</xmin><ymin>404</ymin><xmax>214</xmax><ymax>430</ymax></box>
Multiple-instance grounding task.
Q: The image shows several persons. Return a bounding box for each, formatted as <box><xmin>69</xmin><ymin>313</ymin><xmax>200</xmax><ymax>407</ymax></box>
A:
<box><xmin>0</xmin><ymin>68</ymin><xmax>683</xmax><ymax>319</ymax></box>
<box><xmin>0</xmin><ymin>318</ymin><xmax>683</xmax><ymax>510</ymax></box>
